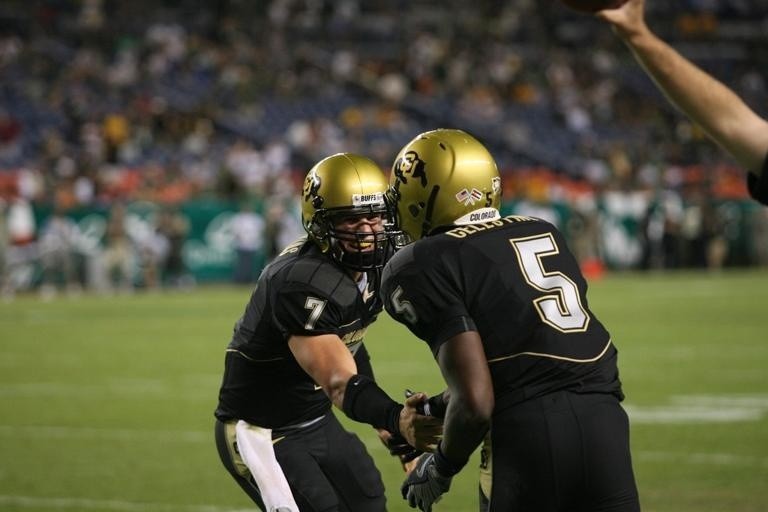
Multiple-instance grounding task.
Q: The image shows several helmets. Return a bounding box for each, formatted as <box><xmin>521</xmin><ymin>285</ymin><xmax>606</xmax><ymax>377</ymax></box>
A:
<box><xmin>301</xmin><ymin>153</ymin><xmax>392</xmax><ymax>271</ymax></box>
<box><xmin>382</xmin><ymin>127</ymin><xmax>503</xmax><ymax>241</ymax></box>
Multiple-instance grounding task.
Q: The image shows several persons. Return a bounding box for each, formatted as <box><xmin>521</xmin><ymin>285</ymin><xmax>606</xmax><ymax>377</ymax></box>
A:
<box><xmin>0</xmin><ymin>0</ymin><xmax>768</xmax><ymax>284</ymax></box>
<box><xmin>214</xmin><ymin>154</ymin><xmax>445</xmax><ymax>511</ymax></box>
<box><xmin>378</xmin><ymin>128</ymin><xmax>641</xmax><ymax>511</ymax></box>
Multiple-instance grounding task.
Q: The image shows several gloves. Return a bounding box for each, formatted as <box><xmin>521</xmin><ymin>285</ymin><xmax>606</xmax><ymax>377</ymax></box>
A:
<box><xmin>391</xmin><ymin>389</ymin><xmax>446</xmax><ymax>464</ymax></box>
<box><xmin>401</xmin><ymin>443</ymin><xmax>454</xmax><ymax>512</ymax></box>
